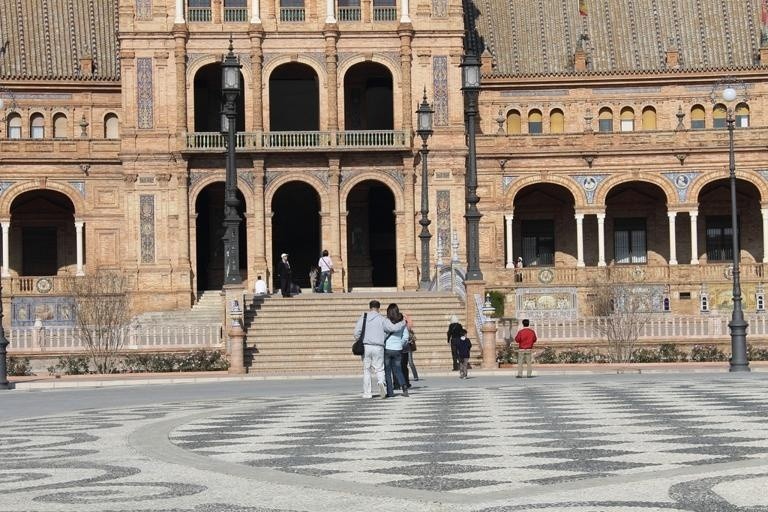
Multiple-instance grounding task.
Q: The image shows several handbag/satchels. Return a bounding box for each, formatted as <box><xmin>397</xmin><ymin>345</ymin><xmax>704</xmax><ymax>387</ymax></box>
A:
<box><xmin>323</xmin><ymin>281</ymin><xmax>328</xmax><ymax>289</ymax></box>
<box><xmin>352</xmin><ymin>339</ymin><xmax>364</xmax><ymax>355</ymax></box>
<box><xmin>330</xmin><ymin>268</ymin><xmax>334</xmax><ymax>274</ymax></box>
<box><xmin>409</xmin><ymin>339</ymin><xmax>416</xmax><ymax>351</ymax></box>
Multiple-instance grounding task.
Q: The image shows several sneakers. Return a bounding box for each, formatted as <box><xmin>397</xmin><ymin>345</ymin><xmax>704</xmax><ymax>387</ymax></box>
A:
<box><xmin>362</xmin><ymin>394</ymin><xmax>372</xmax><ymax>398</ymax></box>
<box><xmin>414</xmin><ymin>377</ymin><xmax>418</xmax><ymax>381</ymax></box>
<box><xmin>516</xmin><ymin>375</ymin><xmax>531</xmax><ymax>378</ymax></box>
<box><xmin>379</xmin><ymin>382</ymin><xmax>411</xmax><ymax>398</ymax></box>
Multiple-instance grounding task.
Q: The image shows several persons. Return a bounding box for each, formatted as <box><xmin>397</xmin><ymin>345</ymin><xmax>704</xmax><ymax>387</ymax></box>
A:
<box><xmin>515</xmin><ymin>319</ymin><xmax>537</xmax><ymax>378</ymax></box>
<box><xmin>255</xmin><ymin>250</ymin><xmax>333</xmax><ymax>297</ymax></box>
<box><xmin>353</xmin><ymin>301</ymin><xmax>472</xmax><ymax>399</ymax></box>
<box><xmin>516</xmin><ymin>257</ymin><xmax>523</xmax><ymax>269</ymax></box>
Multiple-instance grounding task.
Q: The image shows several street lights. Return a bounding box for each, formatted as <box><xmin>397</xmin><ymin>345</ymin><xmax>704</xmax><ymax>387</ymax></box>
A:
<box><xmin>416</xmin><ymin>85</ymin><xmax>435</xmax><ymax>289</ymax></box>
<box><xmin>458</xmin><ymin>28</ymin><xmax>485</xmax><ymax>280</ymax></box>
<box><xmin>219</xmin><ymin>33</ymin><xmax>243</xmax><ymax>285</ymax></box>
<box><xmin>707</xmin><ymin>75</ymin><xmax>752</xmax><ymax>372</ymax></box>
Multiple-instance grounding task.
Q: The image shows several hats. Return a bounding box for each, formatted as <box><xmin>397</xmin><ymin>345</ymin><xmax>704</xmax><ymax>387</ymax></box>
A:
<box><xmin>281</xmin><ymin>253</ymin><xmax>288</xmax><ymax>257</ymax></box>
<box><xmin>450</xmin><ymin>315</ymin><xmax>458</xmax><ymax>323</ymax></box>
